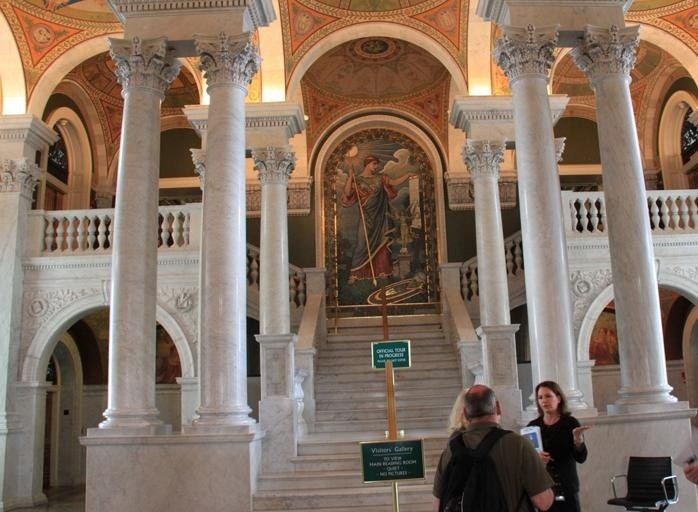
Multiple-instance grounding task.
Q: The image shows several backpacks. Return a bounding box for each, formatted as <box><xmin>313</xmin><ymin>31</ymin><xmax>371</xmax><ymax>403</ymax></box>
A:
<box><xmin>439</xmin><ymin>428</ymin><xmax>514</xmax><ymax>512</ymax></box>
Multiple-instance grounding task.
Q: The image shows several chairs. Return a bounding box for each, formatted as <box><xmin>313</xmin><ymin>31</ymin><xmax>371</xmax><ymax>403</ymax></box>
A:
<box><xmin>608</xmin><ymin>456</ymin><xmax>679</xmax><ymax>512</ymax></box>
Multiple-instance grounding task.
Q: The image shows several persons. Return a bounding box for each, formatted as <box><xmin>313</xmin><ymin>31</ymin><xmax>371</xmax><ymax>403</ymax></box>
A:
<box><xmin>339</xmin><ymin>155</ymin><xmax>417</xmax><ymax>285</ymax></box>
<box><xmin>432</xmin><ymin>382</ymin><xmax>591</xmax><ymax>511</ymax></box>
<box><xmin>683</xmin><ymin>456</ymin><xmax>698</xmax><ymax>483</ymax></box>
